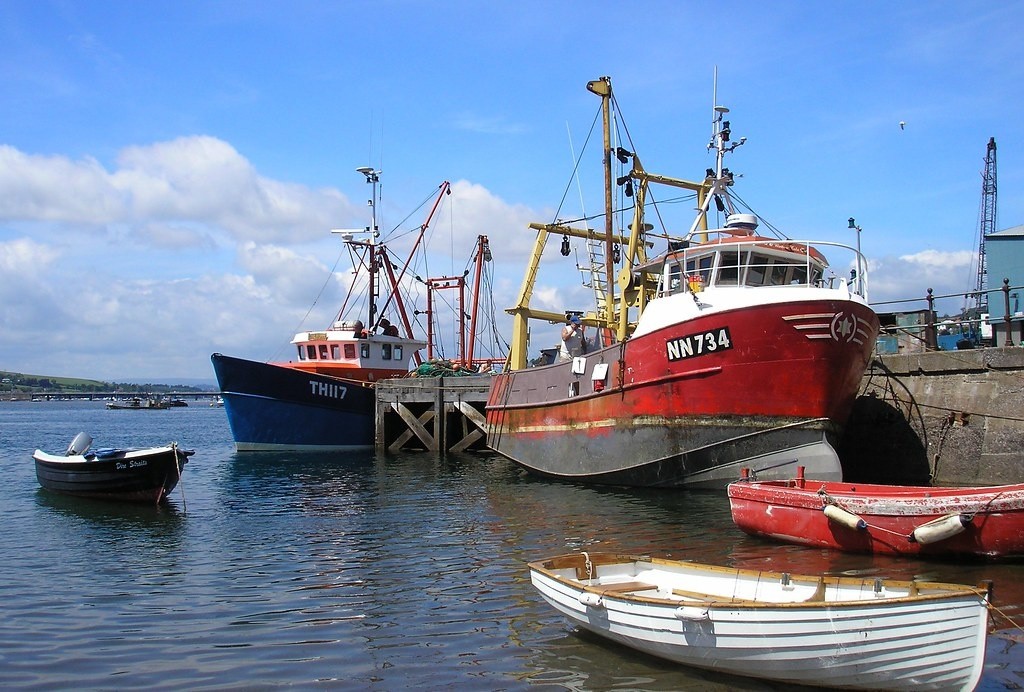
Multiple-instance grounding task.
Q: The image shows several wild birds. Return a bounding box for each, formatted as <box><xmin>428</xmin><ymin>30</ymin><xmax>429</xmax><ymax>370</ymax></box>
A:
<box><xmin>898</xmin><ymin>119</ymin><xmax>908</xmax><ymax>134</ymax></box>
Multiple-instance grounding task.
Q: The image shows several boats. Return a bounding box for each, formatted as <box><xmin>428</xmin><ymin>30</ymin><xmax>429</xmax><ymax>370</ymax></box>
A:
<box><xmin>484</xmin><ymin>62</ymin><xmax>880</xmax><ymax>495</ymax></box>
<box><xmin>210</xmin><ymin>164</ymin><xmax>509</xmax><ymax>455</ymax></box>
<box><xmin>727</xmin><ymin>464</ymin><xmax>1024</xmax><ymax>556</ymax></box>
<box><xmin>524</xmin><ymin>549</ymin><xmax>994</xmax><ymax>692</ymax></box>
<box><xmin>102</xmin><ymin>395</ymin><xmax>225</xmax><ymax>411</ymax></box>
<box><xmin>32</xmin><ymin>431</ymin><xmax>194</xmax><ymax>503</ymax></box>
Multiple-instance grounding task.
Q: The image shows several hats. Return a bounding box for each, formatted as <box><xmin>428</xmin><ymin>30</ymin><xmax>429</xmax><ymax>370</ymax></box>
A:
<box><xmin>570</xmin><ymin>316</ymin><xmax>582</xmax><ymax>324</ymax></box>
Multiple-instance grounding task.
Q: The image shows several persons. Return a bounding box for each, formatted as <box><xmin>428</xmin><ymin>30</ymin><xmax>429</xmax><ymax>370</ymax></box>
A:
<box><xmin>481</xmin><ymin>359</ymin><xmax>492</xmax><ymax>372</ymax></box>
<box><xmin>558</xmin><ymin>316</ymin><xmax>587</xmax><ymax>362</ymax></box>
